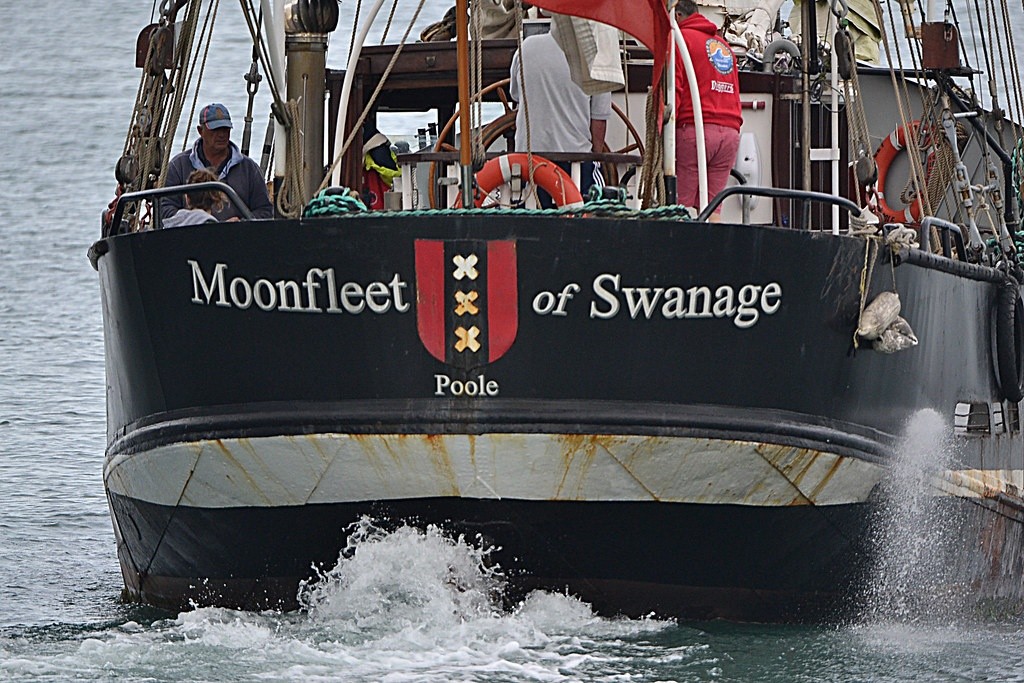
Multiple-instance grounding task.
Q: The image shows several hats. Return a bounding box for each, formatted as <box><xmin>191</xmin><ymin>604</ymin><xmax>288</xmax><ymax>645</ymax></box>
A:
<box><xmin>199</xmin><ymin>102</ymin><xmax>233</xmax><ymax>129</ymax></box>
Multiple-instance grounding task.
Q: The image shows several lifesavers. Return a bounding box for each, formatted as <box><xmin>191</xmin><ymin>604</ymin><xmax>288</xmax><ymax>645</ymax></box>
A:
<box><xmin>996</xmin><ymin>285</ymin><xmax>1024</xmax><ymax>402</ymax></box>
<box><xmin>865</xmin><ymin>119</ymin><xmax>940</xmax><ymax>228</ymax></box>
<box><xmin>449</xmin><ymin>152</ymin><xmax>587</xmax><ymax>218</ymax></box>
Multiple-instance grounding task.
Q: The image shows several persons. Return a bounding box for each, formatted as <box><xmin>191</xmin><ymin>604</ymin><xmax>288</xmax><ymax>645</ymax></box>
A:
<box><xmin>510</xmin><ymin>30</ymin><xmax>611</xmax><ymax>211</ymax></box>
<box><xmin>666</xmin><ymin>0</ymin><xmax>738</xmax><ymax>223</ymax></box>
<box><xmin>157</xmin><ymin>103</ymin><xmax>274</xmax><ymax>230</ymax></box>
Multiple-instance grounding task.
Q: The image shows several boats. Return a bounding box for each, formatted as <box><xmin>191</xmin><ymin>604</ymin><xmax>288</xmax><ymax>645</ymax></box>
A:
<box><xmin>84</xmin><ymin>0</ymin><xmax>1024</xmax><ymax>632</ymax></box>
